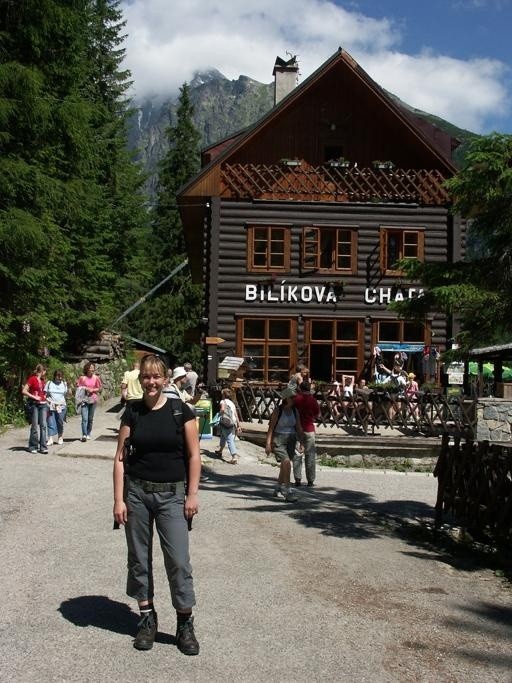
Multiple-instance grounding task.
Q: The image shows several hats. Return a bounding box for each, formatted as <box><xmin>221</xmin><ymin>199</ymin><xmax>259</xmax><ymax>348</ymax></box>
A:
<box><xmin>171</xmin><ymin>366</ymin><xmax>189</xmax><ymax>381</ymax></box>
<box><xmin>281</xmin><ymin>389</ymin><xmax>296</xmax><ymax>400</ymax></box>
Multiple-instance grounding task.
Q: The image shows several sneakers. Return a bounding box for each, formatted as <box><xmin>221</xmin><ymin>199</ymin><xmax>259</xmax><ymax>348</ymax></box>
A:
<box><xmin>28</xmin><ymin>449</ymin><xmax>49</xmax><ymax>456</ymax></box>
<box><xmin>83</xmin><ymin>434</ymin><xmax>91</xmax><ymax>442</ymax></box>
<box><xmin>175</xmin><ymin>612</ymin><xmax>201</xmax><ymax>657</ymax></box>
<box><xmin>293</xmin><ymin>479</ymin><xmax>302</xmax><ymax>488</ymax></box>
<box><xmin>307</xmin><ymin>480</ymin><xmax>318</xmax><ymax>488</ymax></box>
<box><xmin>285</xmin><ymin>492</ymin><xmax>299</xmax><ymax>504</ymax></box>
<box><xmin>131</xmin><ymin>610</ymin><xmax>159</xmax><ymax>650</ymax></box>
<box><xmin>214</xmin><ymin>450</ymin><xmax>240</xmax><ymax>465</ymax></box>
<box><xmin>47</xmin><ymin>438</ymin><xmax>64</xmax><ymax>447</ymax></box>
<box><xmin>272</xmin><ymin>489</ymin><xmax>285</xmax><ymax>499</ymax></box>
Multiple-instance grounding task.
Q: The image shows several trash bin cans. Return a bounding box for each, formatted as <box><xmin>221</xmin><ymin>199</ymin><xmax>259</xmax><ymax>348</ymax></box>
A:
<box><xmin>192</xmin><ymin>397</ymin><xmax>214</xmax><ymax>439</ymax></box>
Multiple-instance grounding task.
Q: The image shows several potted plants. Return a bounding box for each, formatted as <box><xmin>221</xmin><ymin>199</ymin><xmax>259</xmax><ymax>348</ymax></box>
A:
<box><xmin>420</xmin><ymin>382</ymin><xmax>445</xmax><ymax>395</ymax></box>
<box><xmin>366</xmin><ymin>380</ymin><xmax>398</xmax><ymax>395</ymax></box>
<box><xmin>312</xmin><ymin>379</ymin><xmax>337</xmax><ymax>391</ymax></box>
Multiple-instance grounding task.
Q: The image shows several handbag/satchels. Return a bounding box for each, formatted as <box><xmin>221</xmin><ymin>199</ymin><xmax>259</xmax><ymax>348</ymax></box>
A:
<box><xmin>219</xmin><ymin>415</ymin><xmax>234</xmax><ymax>430</ymax></box>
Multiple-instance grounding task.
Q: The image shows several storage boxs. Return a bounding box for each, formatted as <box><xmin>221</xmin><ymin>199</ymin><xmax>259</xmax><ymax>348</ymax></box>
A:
<box><xmin>495</xmin><ymin>382</ymin><xmax>512</xmax><ymax>398</ymax></box>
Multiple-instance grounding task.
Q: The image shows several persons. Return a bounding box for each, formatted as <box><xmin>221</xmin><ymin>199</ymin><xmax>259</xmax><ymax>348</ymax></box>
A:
<box><xmin>119</xmin><ymin>360</ymin><xmax>204</xmax><ymax>414</ymax></box>
<box><xmin>213</xmin><ymin>388</ymin><xmax>242</xmax><ymax>464</ymax></box>
<box><xmin>21</xmin><ymin>363</ymin><xmax>49</xmax><ymax>454</ymax></box>
<box><xmin>112</xmin><ymin>354</ymin><xmax>202</xmax><ymax>656</ymax></box>
<box><xmin>75</xmin><ymin>362</ymin><xmax>101</xmax><ymax>443</ymax></box>
<box><xmin>264</xmin><ymin>387</ymin><xmax>306</xmax><ymax>503</ymax></box>
<box><xmin>289</xmin><ymin>363</ymin><xmax>304</xmax><ymax>388</ymax></box>
<box><xmin>43</xmin><ymin>368</ymin><xmax>68</xmax><ymax>446</ymax></box>
<box><xmin>301</xmin><ymin>368</ymin><xmax>312</xmax><ymax>384</ymax></box>
<box><xmin>327</xmin><ymin>363</ymin><xmax>419</xmax><ymax>421</ymax></box>
<box><xmin>292</xmin><ymin>381</ymin><xmax>319</xmax><ymax>486</ymax></box>
